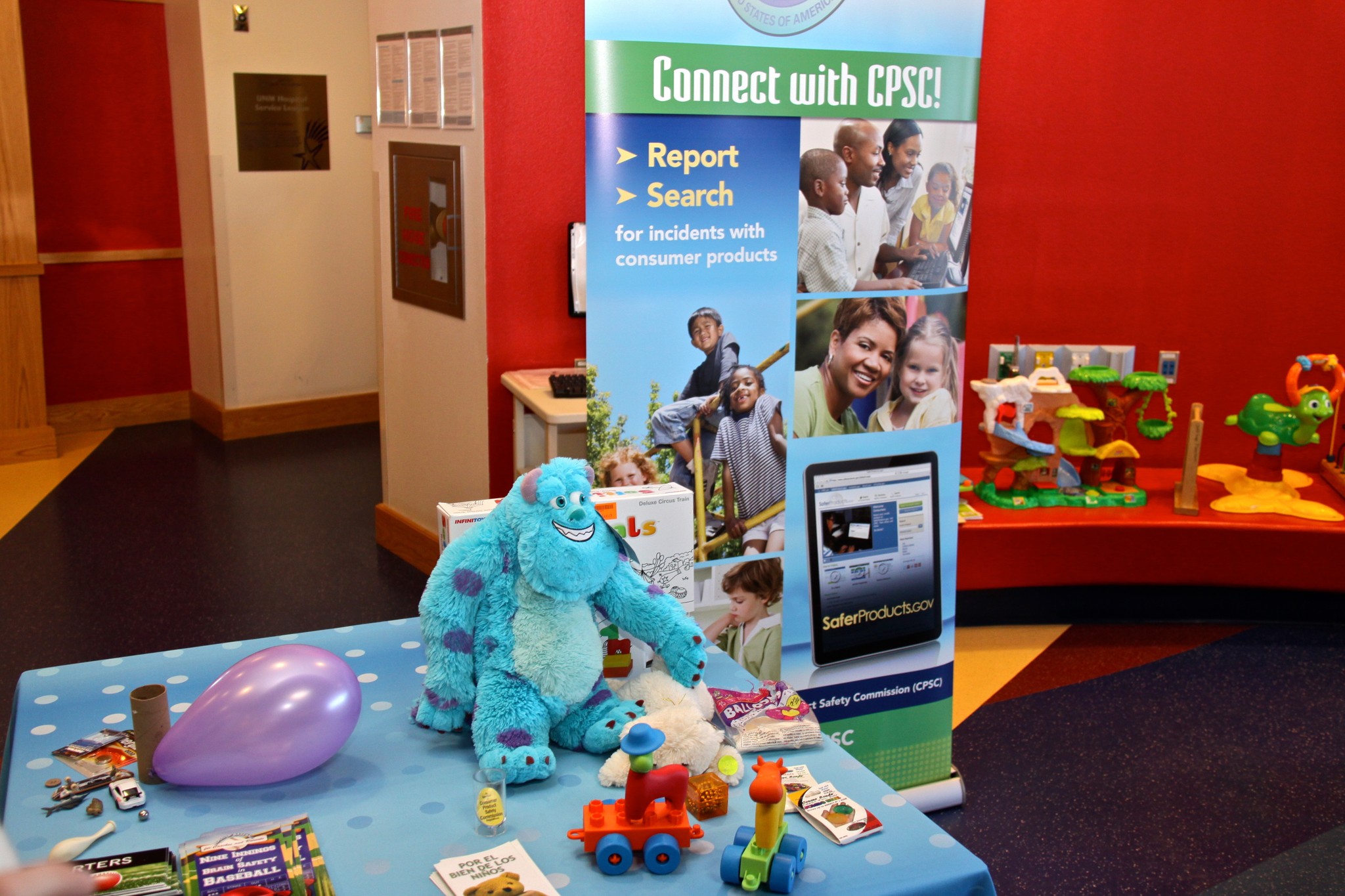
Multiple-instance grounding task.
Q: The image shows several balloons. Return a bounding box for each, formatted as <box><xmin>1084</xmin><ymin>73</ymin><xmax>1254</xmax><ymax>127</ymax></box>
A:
<box><xmin>148</xmin><ymin>644</ymin><xmax>362</xmax><ymax>788</ymax></box>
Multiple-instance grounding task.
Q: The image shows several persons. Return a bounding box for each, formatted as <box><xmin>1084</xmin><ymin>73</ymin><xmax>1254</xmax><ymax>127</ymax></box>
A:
<box><xmin>796</xmin><ymin>148</ymin><xmax>923</xmax><ymax>293</ymax></box>
<box><xmin>864</xmin><ymin>315</ymin><xmax>958</xmax><ymax>432</ymax></box>
<box><xmin>650</xmin><ymin>307</ymin><xmax>741</xmax><ymax>513</ymax></box>
<box><xmin>708</xmin><ymin>366</ymin><xmax>786</xmax><ymax>556</ymax></box>
<box><xmin>831</xmin><ymin>118</ymin><xmax>941</xmax><ymax>281</ymax></box>
<box><xmin>876</xmin><ymin>119</ymin><xmax>922</xmax><ymax>278</ymax></box>
<box><xmin>792</xmin><ymin>296</ymin><xmax>910</xmax><ymax>439</ymax></box>
<box><xmin>699</xmin><ymin>557</ymin><xmax>784</xmax><ymax>684</ymax></box>
<box><xmin>898</xmin><ymin>161</ymin><xmax>960</xmax><ymax>260</ymax></box>
<box><xmin>599</xmin><ymin>448</ymin><xmax>660</xmax><ymax>488</ymax></box>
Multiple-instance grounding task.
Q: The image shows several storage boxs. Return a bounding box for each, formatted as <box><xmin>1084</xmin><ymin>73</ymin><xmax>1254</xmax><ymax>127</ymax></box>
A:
<box><xmin>437</xmin><ymin>479</ymin><xmax>696</xmax><ymax>677</ymax></box>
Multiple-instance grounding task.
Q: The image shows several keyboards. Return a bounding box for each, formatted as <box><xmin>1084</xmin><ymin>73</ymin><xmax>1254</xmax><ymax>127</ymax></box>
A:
<box><xmin>908</xmin><ymin>251</ymin><xmax>947</xmax><ymax>289</ymax></box>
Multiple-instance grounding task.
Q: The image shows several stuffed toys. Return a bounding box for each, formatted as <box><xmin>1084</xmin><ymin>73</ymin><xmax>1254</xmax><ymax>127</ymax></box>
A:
<box><xmin>596</xmin><ymin>654</ymin><xmax>745</xmax><ymax>790</ymax></box>
<box><xmin>410</xmin><ymin>456</ymin><xmax>708</xmax><ymax>783</ymax></box>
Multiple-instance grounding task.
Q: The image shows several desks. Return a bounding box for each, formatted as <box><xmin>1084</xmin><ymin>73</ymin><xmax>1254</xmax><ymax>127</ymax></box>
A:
<box><xmin>957</xmin><ymin>465</ymin><xmax>1345</xmax><ymax>593</ymax></box>
<box><xmin>0</xmin><ymin>616</ymin><xmax>1003</xmax><ymax>895</ymax></box>
<box><xmin>500</xmin><ymin>368</ymin><xmax>586</xmax><ymax>494</ymax></box>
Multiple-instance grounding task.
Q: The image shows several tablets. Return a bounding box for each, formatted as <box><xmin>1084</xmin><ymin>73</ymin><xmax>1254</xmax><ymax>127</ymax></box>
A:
<box><xmin>804</xmin><ymin>450</ymin><xmax>942</xmax><ymax>666</ymax></box>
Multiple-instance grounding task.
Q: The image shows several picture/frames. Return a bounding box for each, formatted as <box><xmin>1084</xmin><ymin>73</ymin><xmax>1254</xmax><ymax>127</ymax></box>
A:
<box><xmin>388</xmin><ymin>140</ymin><xmax>467</xmax><ymax>321</ymax></box>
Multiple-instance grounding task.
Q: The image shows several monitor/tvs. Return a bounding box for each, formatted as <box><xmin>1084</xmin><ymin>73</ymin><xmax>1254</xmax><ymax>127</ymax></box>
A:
<box><xmin>948</xmin><ymin>183</ymin><xmax>973</xmax><ymax>287</ymax></box>
